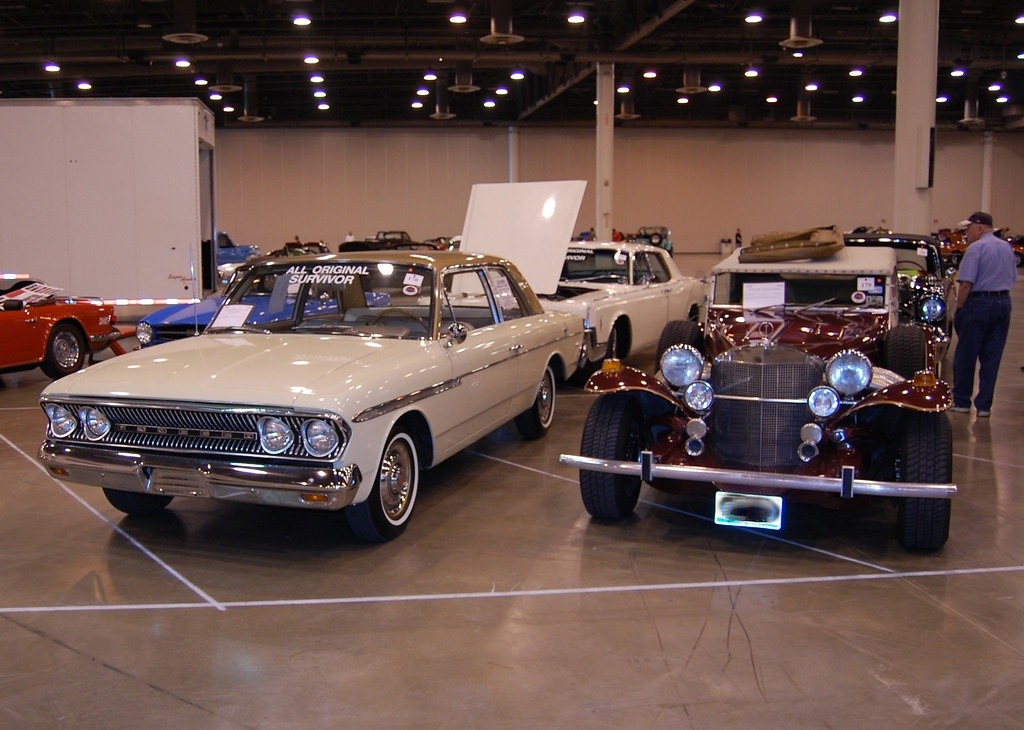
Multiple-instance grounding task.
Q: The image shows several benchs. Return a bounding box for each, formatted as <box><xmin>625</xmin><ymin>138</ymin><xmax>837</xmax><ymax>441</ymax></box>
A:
<box><xmin>345</xmin><ymin>306</ymin><xmax>499</xmax><ymax>330</ymax></box>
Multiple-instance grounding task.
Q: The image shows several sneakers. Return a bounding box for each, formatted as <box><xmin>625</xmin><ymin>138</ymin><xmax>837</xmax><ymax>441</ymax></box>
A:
<box><xmin>949</xmin><ymin>403</ymin><xmax>971</xmax><ymax>412</ymax></box>
<box><xmin>976</xmin><ymin>409</ymin><xmax>991</xmax><ymax>417</ymax></box>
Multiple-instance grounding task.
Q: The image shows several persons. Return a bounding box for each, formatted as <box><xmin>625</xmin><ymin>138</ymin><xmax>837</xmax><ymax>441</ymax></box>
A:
<box><xmin>293</xmin><ymin>236</ymin><xmax>300</xmax><ymax>242</ymax></box>
<box><xmin>727</xmin><ymin>228</ymin><xmax>742</xmax><ymax>259</ymax></box>
<box><xmin>586</xmin><ymin>228</ymin><xmax>596</xmax><ymax>241</ymax></box>
<box><xmin>345</xmin><ymin>232</ymin><xmax>354</xmax><ymax>242</ymax></box>
<box><xmin>949</xmin><ymin>211</ymin><xmax>1017</xmax><ymax>417</ymax></box>
<box><xmin>612</xmin><ymin>228</ymin><xmax>625</xmax><ymax>241</ymax></box>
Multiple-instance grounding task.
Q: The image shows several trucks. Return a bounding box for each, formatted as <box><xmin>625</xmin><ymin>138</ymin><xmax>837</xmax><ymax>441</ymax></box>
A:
<box><xmin>217</xmin><ymin>231</ymin><xmax>259</xmax><ymax>264</ymax></box>
<box><xmin>623</xmin><ymin>226</ymin><xmax>674</xmax><ymax>257</ymax></box>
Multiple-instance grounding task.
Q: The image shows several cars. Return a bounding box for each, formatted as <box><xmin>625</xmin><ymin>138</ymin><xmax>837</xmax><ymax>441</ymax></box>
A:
<box><xmin>841</xmin><ymin>233</ymin><xmax>956</xmax><ymax>349</ymax></box>
<box><xmin>339</xmin><ymin>230</ymin><xmax>439</xmax><ymax>275</ymax></box>
<box><xmin>133</xmin><ymin>263</ymin><xmax>393</xmax><ymax>350</ymax></box>
<box><xmin>558</xmin><ymin>247</ymin><xmax>957</xmax><ymax>553</ymax></box>
<box><xmin>217</xmin><ymin>241</ymin><xmax>333</xmax><ymax>295</ymax></box>
<box><xmin>417</xmin><ymin>240</ymin><xmax>705</xmax><ymax>385</ymax></box>
<box><xmin>939</xmin><ymin>227</ymin><xmax>1024</xmax><ymax>269</ymax></box>
<box><xmin>0</xmin><ymin>274</ymin><xmax>121</xmax><ymax>379</ymax></box>
<box><xmin>39</xmin><ymin>250</ymin><xmax>584</xmax><ymax>542</ymax></box>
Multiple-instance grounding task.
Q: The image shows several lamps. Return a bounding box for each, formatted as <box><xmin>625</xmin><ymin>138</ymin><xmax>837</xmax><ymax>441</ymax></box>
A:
<box><xmin>238</xmin><ymin>101</ymin><xmax>264</xmax><ymax>122</ymax></box>
<box><xmin>447</xmin><ymin>67</ymin><xmax>480</xmax><ymax>92</ymax></box>
<box><xmin>479</xmin><ymin>10</ymin><xmax>524</xmax><ymax>45</ymax></box>
<box><xmin>160</xmin><ymin>15</ymin><xmax>208</xmax><ymax>42</ymax></box>
<box><xmin>430</xmin><ymin>84</ymin><xmax>457</xmax><ymax>119</ymax></box>
<box><xmin>791</xmin><ymin>97</ymin><xmax>817</xmax><ymax>121</ymax></box>
<box><xmin>615</xmin><ymin>101</ymin><xmax>640</xmax><ymax>119</ymax></box>
<box><xmin>208</xmin><ymin>76</ymin><xmax>243</xmax><ymax>93</ymax></box>
<box><xmin>959</xmin><ymin>98</ymin><xmax>982</xmax><ymax>124</ymax></box>
<box><xmin>778</xmin><ymin>12</ymin><xmax>823</xmax><ymax>48</ymax></box>
<box><xmin>676</xmin><ymin>69</ymin><xmax>707</xmax><ymax>94</ymax></box>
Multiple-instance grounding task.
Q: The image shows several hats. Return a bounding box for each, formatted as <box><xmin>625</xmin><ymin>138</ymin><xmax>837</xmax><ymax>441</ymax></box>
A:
<box><xmin>958</xmin><ymin>211</ymin><xmax>993</xmax><ymax>226</ymax></box>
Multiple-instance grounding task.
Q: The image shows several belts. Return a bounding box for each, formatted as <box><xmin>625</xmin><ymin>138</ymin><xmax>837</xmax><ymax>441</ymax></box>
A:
<box><xmin>969</xmin><ymin>290</ymin><xmax>1010</xmax><ymax>297</ymax></box>
